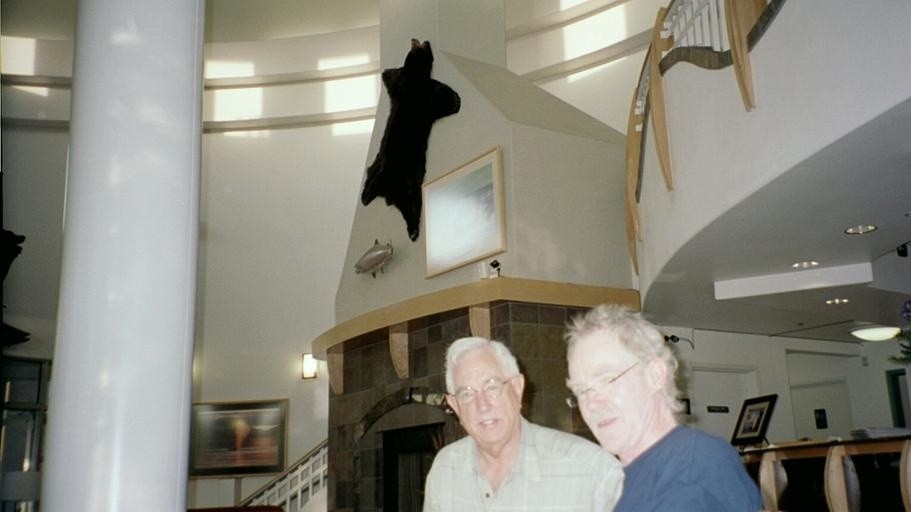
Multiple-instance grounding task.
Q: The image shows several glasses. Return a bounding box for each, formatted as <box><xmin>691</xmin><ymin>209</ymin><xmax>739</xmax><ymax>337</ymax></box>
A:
<box><xmin>563</xmin><ymin>358</ymin><xmax>650</xmax><ymax>408</ymax></box>
<box><xmin>450</xmin><ymin>373</ymin><xmax>517</xmax><ymax>404</ymax></box>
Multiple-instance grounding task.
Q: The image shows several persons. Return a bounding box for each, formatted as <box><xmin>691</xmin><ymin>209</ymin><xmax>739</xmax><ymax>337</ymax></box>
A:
<box><xmin>564</xmin><ymin>303</ymin><xmax>763</xmax><ymax>512</ymax></box>
<box><xmin>422</xmin><ymin>336</ymin><xmax>625</xmax><ymax>512</ymax></box>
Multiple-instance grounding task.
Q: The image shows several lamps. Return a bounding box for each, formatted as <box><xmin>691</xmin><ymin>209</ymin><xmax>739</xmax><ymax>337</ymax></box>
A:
<box><xmin>848</xmin><ymin>324</ymin><xmax>902</xmax><ymax>344</ymax></box>
<box><xmin>301</xmin><ymin>352</ymin><xmax>317</xmax><ymax>381</ymax></box>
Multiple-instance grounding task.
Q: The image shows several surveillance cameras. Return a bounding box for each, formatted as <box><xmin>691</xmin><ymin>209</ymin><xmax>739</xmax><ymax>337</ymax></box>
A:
<box><xmin>670</xmin><ymin>335</ymin><xmax>680</xmax><ymax>343</ymax></box>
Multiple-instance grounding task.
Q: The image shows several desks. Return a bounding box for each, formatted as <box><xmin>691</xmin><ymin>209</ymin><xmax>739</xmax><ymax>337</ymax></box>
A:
<box><xmin>737</xmin><ymin>433</ymin><xmax>911</xmax><ymax>512</ymax></box>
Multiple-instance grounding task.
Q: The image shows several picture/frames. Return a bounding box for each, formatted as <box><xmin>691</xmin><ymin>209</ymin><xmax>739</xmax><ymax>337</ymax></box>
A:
<box><xmin>418</xmin><ymin>143</ymin><xmax>507</xmax><ymax>281</ymax></box>
<box><xmin>189</xmin><ymin>396</ymin><xmax>290</xmax><ymax>481</ymax></box>
<box><xmin>730</xmin><ymin>393</ymin><xmax>778</xmax><ymax>446</ymax></box>
